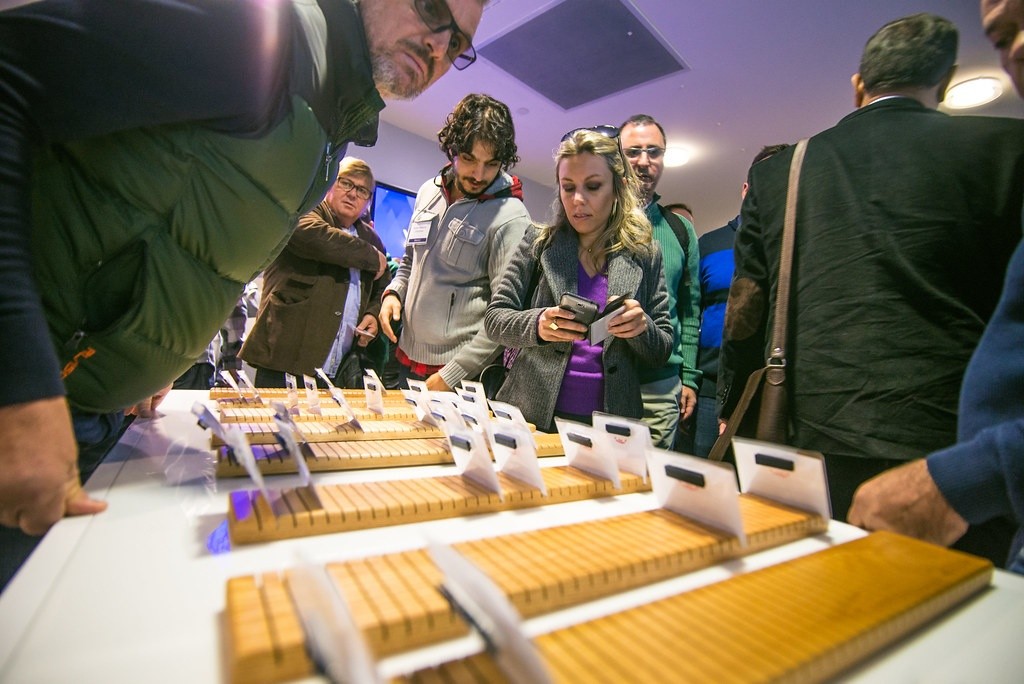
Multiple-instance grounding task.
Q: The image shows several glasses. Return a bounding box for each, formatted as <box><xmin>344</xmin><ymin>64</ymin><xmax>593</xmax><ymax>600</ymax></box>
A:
<box><xmin>623</xmin><ymin>147</ymin><xmax>665</xmax><ymax>161</ymax></box>
<box><xmin>414</xmin><ymin>0</ymin><xmax>477</xmax><ymax>70</ymax></box>
<box><xmin>336</xmin><ymin>175</ymin><xmax>373</xmax><ymax>200</ymax></box>
<box><xmin>561</xmin><ymin>124</ymin><xmax>626</xmax><ymax>177</ymax></box>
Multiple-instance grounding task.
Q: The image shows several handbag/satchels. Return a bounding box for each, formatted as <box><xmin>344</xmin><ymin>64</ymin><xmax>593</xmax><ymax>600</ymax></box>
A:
<box><xmin>334</xmin><ymin>334</ymin><xmax>387</xmax><ymax>389</ymax></box>
<box><xmin>709</xmin><ymin>358</ymin><xmax>792</xmax><ymax>461</ymax></box>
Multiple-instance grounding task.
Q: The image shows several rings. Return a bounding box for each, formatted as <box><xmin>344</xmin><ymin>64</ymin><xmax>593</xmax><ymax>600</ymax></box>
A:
<box><xmin>549</xmin><ymin>318</ymin><xmax>559</xmax><ymax>332</ymax></box>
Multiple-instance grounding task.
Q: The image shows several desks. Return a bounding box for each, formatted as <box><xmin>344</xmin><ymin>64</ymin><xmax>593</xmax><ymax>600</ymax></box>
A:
<box><xmin>0</xmin><ymin>388</ymin><xmax>1024</xmax><ymax>684</ymax></box>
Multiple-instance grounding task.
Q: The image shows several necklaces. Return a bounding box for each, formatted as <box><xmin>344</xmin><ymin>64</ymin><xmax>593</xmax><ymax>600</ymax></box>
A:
<box><xmin>580</xmin><ymin>241</ymin><xmax>600</xmax><ymax>254</ymax></box>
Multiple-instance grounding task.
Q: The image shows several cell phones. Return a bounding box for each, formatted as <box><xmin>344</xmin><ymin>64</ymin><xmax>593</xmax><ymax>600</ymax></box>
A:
<box><xmin>560</xmin><ymin>292</ymin><xmax>599</xmax><ymax>342</ymax></box>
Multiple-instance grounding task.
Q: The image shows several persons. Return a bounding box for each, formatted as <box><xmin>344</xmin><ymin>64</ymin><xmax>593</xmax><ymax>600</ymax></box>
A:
<box><xmin>175</xmin><ymin>92</ymin><xmax>794</xmax><ymax>463</ymax></box>
<box><xmin>0</xmin><ymin>0</ymin><xmax>489</xmax><ymax>603</ymax></box>
<box><xmin>716</xmin><ymin>0</ymin><xmax>1024</xmax><ymax>577</ymax></box>
